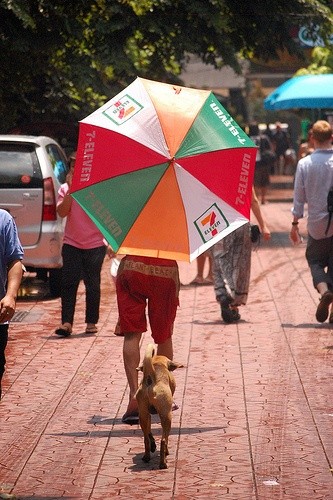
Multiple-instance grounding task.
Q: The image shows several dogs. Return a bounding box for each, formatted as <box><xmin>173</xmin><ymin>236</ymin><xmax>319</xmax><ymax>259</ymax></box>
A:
<box><xmin>135</xmin><ymin>344</ymin><xmax>184</xmax><ymax>469</ymax></box>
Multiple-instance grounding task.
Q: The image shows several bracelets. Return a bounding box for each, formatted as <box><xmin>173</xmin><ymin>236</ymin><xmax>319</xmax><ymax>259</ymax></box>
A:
<box><xmin>291</xmin><ymin>222</ymin><xmax>299</xmax><ymax>225</ymax></box>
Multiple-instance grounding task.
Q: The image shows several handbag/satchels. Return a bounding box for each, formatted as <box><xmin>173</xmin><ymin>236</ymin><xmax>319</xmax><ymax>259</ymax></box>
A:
<box><xmin>262</xmin><ymin>151</ymin><xmax>276</xmax><ymax>164</ymax></box>
<box><xmin>251</xmin><ymin>225</ymin><xmax>260</xmax><ymax>244</ymax></box>
<box><xmin>325</xmin><ymin>185</ymin><xmax>332</xmax><ymax>235</ymax></box>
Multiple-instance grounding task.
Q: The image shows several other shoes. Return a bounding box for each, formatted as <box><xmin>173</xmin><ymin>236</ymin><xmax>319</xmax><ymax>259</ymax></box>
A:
<box><xmin>220</xmin><ymin>301</ymin><xmax>241</xmax><ymax>323</ymax></box>
<box><xmin>189</xmin><ymin>276</ymin><xmax>214</xmax><ymax>285</ymax></box>
<box><xmin>316</xmin><ymin>290</ymin><xmax>333</xmax><ymax>324</ymax></box>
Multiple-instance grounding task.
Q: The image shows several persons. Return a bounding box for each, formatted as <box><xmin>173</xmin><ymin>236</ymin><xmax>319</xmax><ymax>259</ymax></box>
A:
<box><xmin>115</xmin><ymin>255</ymin><xmax>179</xmax><ymax>422</ymax></box>
<box><xmin>188</xmin><ymin>101</ymin><xmax>333</xmax><ymax>328</ymax></box>
<box><xmin>0</xmin><ymin>208</ymin><xmax>25</xmax><ymax>406</ymax></box>
<box><xmin>55</xmin><ymin>151</ymin><xmax>116</xmax><ymax>337</ymax></box>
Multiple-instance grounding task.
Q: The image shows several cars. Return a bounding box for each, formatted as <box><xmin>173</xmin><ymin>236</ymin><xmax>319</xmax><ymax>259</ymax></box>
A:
<box><xmin>1</xmin><ymin>133</ymin><xmax>72</xmax><ymax>295</ymax></box>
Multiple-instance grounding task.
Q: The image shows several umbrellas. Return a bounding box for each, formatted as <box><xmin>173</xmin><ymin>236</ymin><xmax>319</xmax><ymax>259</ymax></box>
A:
<box><xmin>263</xmin><ymin>73</ymin><xmax>333</xmax><ymax>111</ymax></box>
<box><xmin>66</xmin><ymin>75</ymin><xmax>261</xmax><ymax>265</ymax></box>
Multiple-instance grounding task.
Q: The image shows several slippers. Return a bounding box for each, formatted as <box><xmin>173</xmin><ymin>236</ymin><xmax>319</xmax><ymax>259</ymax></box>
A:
<box><xmin>171</xmin><ymin>400</ymin><xmax>179</xmax><ymax>410</ymax></box>
<box><xmin>55</xmin><ymin>324</ymin><xmax>72</xmax><ymax>336</ymax></box>
<box><xmin>86</xmin><ymin>326</ymin><xmax>98</xmax><ymax>333</ymax></box>
<box><xmin>121</xmin><ymin>412</ymin><xmax>139</xmax><ymax>424</ymax></box>
